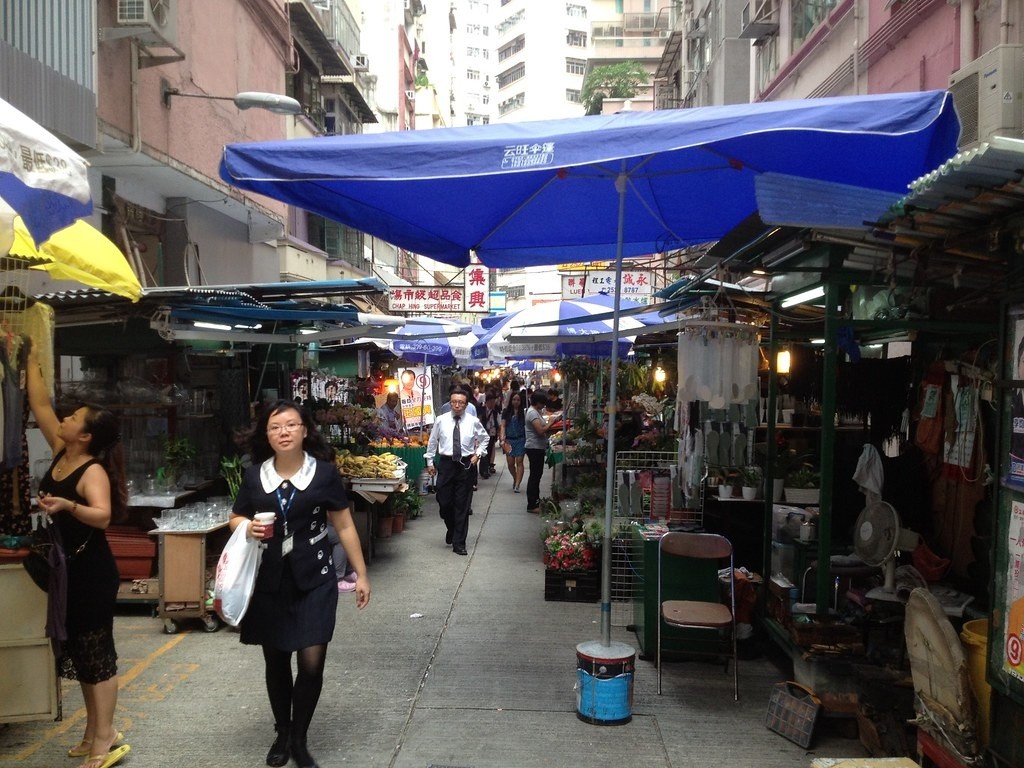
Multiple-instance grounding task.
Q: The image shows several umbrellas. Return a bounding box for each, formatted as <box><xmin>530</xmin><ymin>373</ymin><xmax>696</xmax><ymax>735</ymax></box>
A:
<box><xmin>350</xmin><ymin>290</ymin><xmax>687</xmax><ymax>440</ymax></box>
<box><xmin>217</xmin><ymin>88</ymin><xmax>961</xmax><ymax>645</ymax></box>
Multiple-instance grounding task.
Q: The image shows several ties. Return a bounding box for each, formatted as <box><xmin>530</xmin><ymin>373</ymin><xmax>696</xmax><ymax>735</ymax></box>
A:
<box><xmin>453</xmin><ymin>415</ymin><xmax>461</xmax><ymax>461</ymax></box>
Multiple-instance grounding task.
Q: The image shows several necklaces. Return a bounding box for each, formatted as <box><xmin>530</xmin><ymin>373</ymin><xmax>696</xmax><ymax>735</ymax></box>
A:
<box><xmin>58</xmin><ymin>455</ymin><xmax>92</xmax><ymax>472</ymax></box>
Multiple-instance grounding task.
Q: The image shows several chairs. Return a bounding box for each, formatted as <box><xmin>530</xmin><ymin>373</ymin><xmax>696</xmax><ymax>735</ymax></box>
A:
<box><xmin>656</xmin><ymin>532</ymin><xmax>738</xmax><ymax>701</ymax></box>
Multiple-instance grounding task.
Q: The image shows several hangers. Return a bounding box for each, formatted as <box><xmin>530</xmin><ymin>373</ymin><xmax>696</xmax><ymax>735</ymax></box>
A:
<box><xmin>0</xmin><ymin>281</ymin><xmax>28</xmax><ymax>298</ymax></box>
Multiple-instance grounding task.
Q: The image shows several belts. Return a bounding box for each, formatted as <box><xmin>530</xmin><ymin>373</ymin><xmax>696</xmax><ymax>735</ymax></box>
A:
<box><xmin>441</xmin><ymin>456</ymin><xmax>470</xmax><ymax>462</ymax></box>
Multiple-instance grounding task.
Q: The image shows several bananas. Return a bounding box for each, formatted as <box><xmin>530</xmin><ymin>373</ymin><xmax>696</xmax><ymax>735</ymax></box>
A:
<box><xmin>335</xmin><ymin>452</ymin><xmax>400</xmax><ymax>478</ymax></box>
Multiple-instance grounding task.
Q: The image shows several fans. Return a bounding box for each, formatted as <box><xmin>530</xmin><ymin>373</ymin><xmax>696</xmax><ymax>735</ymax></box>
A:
<box><xmin>853</xmin><ymin>501</ymin><xmax>908</xmax><ymax>604</ymax></box>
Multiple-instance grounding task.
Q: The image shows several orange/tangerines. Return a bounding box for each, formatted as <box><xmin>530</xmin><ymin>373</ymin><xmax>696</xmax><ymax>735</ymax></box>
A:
<box><xmin>370</xmin><ymin>433</ymin><xmax>429</xmax><ymax>447</ymax></box>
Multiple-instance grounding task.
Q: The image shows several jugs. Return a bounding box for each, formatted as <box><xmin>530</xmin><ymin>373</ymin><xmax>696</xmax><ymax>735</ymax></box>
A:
<box><xmin>126</xmin><ymin>479</ymin><xmax>177</xmax><ymax>497</ymax></box>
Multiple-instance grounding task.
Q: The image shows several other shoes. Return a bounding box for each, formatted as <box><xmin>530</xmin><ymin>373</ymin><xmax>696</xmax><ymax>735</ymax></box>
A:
<box><xmin>513</xmin><ymin>484</ymin><xmax>515</xmax><ymax>489</ymax></box>
<box><xmin>291</xmin><ymin>748</ymin><xmax>318</xmax><ymax>768</ymax></box>
<box><xmin>514</xmin><ymin>487</ymin><xmax>520</xmax><ymax>492</ymax></box>
<box><xmin>469</xmin><ymin>508</ymin><xmax>472</xmax><ymax>514</ymax></box>
<box><xmin>266</xmin><ymin>721</ymin><xmax>291</xmax><ymax>767</ymax></box>
<box><xmin>489</xmin><ymin>468</ymin><xmax>496</xmax><ymax>473</ymax></box>
<box><xmin>473</xmin><ymin>485</ymin><xmax>477</xmax><ymax>490</ymax></box>
<box><xmin>481</xmin><ymin>473</ymin><xmax>491</xmax><ymax>479</ymax></box>
<box><xmin>489</xmin><ymin>463</ymin><xmax>495</xmax><ymax>467</ymax></box>
<box><xmin>527</xmin><ymin>507</ymin><xmax>539</xmax><ymax>512</ymax></box>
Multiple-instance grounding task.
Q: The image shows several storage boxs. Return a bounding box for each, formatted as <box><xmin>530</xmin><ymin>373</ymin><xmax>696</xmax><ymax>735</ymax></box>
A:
<box><xmin>769</xmin><ymin>542</ymin><xmax>807</xmax><ymax>582</ymax></box>
<box><xmin>766</xmin><ymin>581</ymin><xmax>794</xmax><ymax>622</ymax></box>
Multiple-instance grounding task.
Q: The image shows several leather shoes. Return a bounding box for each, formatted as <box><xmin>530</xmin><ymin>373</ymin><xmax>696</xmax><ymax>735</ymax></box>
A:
<box><xmin>445</xmin><ymin>530</ymin><xmax>453</xmax><ymax>544</ymax></box>
<box><xmin>453</xmin><ymin>546</ymin><xmax>467</xmax><ymax>555</ymax></box>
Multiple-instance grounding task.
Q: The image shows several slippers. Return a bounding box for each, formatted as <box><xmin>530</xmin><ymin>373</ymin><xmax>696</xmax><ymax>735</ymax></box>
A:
<box><xmin>68</xmin><ymin>732</ymin><xmax>123</xmax><ymax>756</ymax></box>
<box><xmin>76</xmin><ymin>744</ymin><xmax>131</xmax><ymax>768</ymax></box>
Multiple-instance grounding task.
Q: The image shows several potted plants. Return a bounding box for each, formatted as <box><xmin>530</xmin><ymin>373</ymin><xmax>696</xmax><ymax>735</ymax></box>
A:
<box><xmin>377</xmin><ymin>479</ymin><xmax>423</xmax><ymax>538</ymax></box>
<box><xmin>764</xmin><ymin>455</ymin><xmax>783</xmax><ymax>504</ymax></box>
<box><xmin>784</xmin><ymin>468</ymin><xmax>820</xmax><ymax>503</ymax></box>
<box><xmin>742</xmin><ymin>469</ymin><xmax>759</xmax><ymax>499</ymax></box>
<box><xmin>718</xmin><ymin>472</ymin><xmax>731</xmax><ymax>499</ymax></box>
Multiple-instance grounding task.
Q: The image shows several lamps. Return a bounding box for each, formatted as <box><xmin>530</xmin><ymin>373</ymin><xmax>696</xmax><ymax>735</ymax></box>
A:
<box><xmin>164</xmin><ymin>86</ymin><xmax>301</xmax><ymax>117</ymax></box>
<box><xmin>778</xmin><ymin>285</ymin><xmax>824</xmax><ymax>311</ymax></box>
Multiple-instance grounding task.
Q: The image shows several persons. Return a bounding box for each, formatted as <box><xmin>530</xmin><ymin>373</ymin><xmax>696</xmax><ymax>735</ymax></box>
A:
<box><xmin>426</xmin><ymin>390</ymin><xmax>490</xmax><ymax>555</ymax></box>
<box><xmin>377</xmin><ymin>392</ymin><xmax>400</xmax><ymax>429</ymax></box>
<box><xmin>27</xmin><ymin>335</ymin><xmax>120</xmax><ymax>768</ymax></box>
<box><xmin>524</xmin><ymin>390</ymin><xmax>563</xmax><ymax>514</ymax></box>
<box><xmin>366</xmin><ymin>394</ymin><xmax>386</xmax><ymax>428</ymax></box>
<box><xmin>229</xmin><ymin>398</ymin><xmax>370</xmax><ymax>768</ymax></box>
<box><xmin>400</xmin><ymin>370</ymin><xmax>421</xmax><ymax>399</ymax></box>
<box><xmin>500</xmin><ymin>392</ymin><xmax>526</xmax><ymax>493</ymax></box>
<box><xmin>441</xmin><ymin>378</ymin><xmax>562</xmax><ymax>515</ymax></box>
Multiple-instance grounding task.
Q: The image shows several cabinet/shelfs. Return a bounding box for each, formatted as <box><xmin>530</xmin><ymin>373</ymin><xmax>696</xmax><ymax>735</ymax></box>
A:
<box><xmin>624</xmin><ymin>522</ymin><xmax>720</xmax><ymax>664</ymax></box>
<box><xmin>149</xmin><ymin>515</ymin><xmax>232</xmax><ymax>632</ymax></box>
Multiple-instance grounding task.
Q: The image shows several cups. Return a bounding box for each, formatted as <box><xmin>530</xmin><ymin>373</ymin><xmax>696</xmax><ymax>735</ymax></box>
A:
<box><xmin>156</xmin><ymin>501</ymin><xmax>227</xmax><ymax>528</ymax></box>
<box><xmin>254</xmin><ymin>511</ymin><xmax>275</xmax><ymax>538</ymax></box>
<box><xmin>800</xmin><ymin>521</ymin><xmax>815</xmax><ymax>541</ymax></box>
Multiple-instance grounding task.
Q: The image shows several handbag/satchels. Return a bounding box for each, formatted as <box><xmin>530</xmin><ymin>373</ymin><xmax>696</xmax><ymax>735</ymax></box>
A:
<box><xmin>23</xmin><ymin>540</ymin><xmax>69</xmax><ymax>594</ymax></box>
<box><xmin>212</xmin><ymin>520</ymin><xmax>269</xmax><ymax>627</ymax></box>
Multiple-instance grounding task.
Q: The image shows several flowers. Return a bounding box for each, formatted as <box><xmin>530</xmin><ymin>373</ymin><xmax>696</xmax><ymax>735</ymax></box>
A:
<box><xmin>546</xmin><ymin>536</ymin><xmax>594</xmax><ymax>571</ymax></box>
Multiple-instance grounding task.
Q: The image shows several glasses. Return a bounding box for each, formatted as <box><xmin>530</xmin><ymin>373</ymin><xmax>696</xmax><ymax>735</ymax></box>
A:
<box><xmin>266</xmin><ymin>422</ymin><xmax>303</xmax><ymax>435</ymax></box>
<box><xmin>450</xmin><ymin>400</ymin><xmax>466</xmax><ymax>407</ymax></box>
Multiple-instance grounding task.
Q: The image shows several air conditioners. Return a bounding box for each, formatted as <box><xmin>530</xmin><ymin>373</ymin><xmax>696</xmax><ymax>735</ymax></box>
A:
<box><xmin>350</xmin><ymin>54</ymin><xmax>370</xmax><ymax>73</ymax></box>
<box><xmin>405</xmin><ymin>90</ymin><xmax>415</xmax><ymax>104</ymax></box>
<box><xmin>117</xmin><ymin>0</ymin><xmax>177</xmax><ymax>49</ymax></box>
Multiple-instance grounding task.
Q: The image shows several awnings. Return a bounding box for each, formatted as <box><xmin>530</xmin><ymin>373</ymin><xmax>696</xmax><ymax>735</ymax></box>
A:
<box><xmin>30</xmin><ymin>277</ymin><xmax>406</xmax><ymax>401</ymax></box>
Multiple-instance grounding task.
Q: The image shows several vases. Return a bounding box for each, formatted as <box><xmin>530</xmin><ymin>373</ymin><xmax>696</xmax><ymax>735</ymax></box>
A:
<box><xmin>545</xmin><ymin>569</ymin><xmax>600</xmax><ymax>605</ymax></box>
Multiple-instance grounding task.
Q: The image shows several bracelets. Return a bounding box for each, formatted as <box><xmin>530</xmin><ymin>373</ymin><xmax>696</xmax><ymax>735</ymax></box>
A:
<box><xmin>70</xmin><ymin>501</ymin><xmax>77</xmax><ymax>514</ymax></box>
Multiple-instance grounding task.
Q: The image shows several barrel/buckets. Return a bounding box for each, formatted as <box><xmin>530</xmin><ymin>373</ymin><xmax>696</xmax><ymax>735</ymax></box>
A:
<box><xmin>961</xmin><ymin>618</ymin><xmax>992</xmax><ymax>747</ymax></box>
<box><xmin>573</xmin><ymin>640</ymin><xmax>635</xmax><ymax>725</ymax></box>
<box><xmin>418</xmin><ymin>473</ymin><xmax>431</xmax><ymax>496</ymax></box>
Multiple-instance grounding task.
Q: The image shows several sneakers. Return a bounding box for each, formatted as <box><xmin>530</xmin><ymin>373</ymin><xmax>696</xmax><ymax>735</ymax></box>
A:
<box><xmin>337</xmin><ymin>580</ymin><xmax>356</xmax><ymax>592</ymax></box>
<box><xmin>343</xmin><ymin>571</ymin><xmax>358</xmax><ymax>581</ymax></box>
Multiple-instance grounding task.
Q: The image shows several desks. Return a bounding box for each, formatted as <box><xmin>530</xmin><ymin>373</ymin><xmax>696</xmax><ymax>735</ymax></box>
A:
<box><xmin>116</xmin><ymin>484</ymin><xmax>195</xmax><ymax>619</ymax></box>
